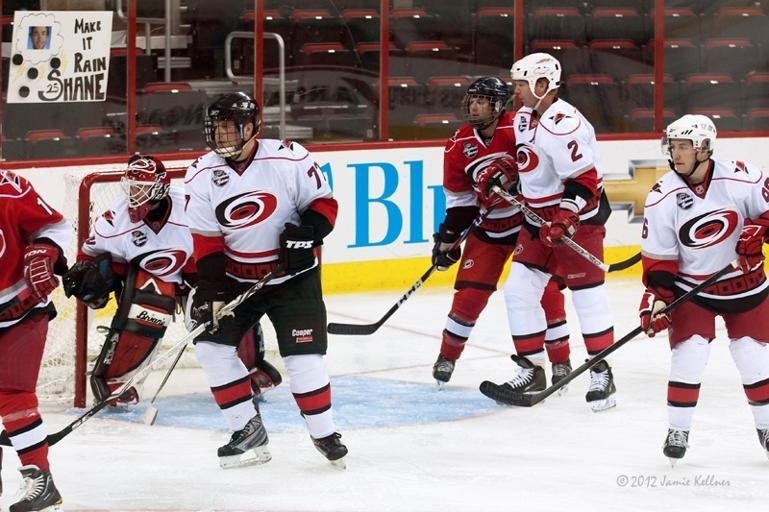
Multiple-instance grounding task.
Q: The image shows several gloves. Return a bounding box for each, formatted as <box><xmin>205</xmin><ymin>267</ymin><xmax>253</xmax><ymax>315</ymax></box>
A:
<box><xmin>476</xmin><ymin>155</ymin><xmax>518</xmax><ymax>208</ymax></box>
<box><xmin>538</xmin><ymin>178</ymin><xmax>595</xmax><ymax>250</ymax></box>
<box><xmin>19</xmin><ymin>242</ymin><xmax>63</xmax><ymax>307</ymax></box>
<box><xmin>183</xmin><ymin>252</ymin><xmax>234</xmax><ymax>341</ymax></box>
<box><xmin>639</xmin><ymin>269</ymin><xmax>676</xmax><ymax>338</ymax></box>
<box><xmin>62</xmin><ymin>257</ymin><xmax>112</xmax><ymax>310</ymax></box>
<box><xmin>736</xmin><ymin>210</ymin><xmax>769</xmax><ymax>276</ymax></box>
<box><xmin>430</xmin><ymin>222</ymin><xmax>461</xmax><ymax>272</ymax></box>
<box><xmin>279</xmin><ymin>221</ymin><xmax>324</xmax><ymax>276</ymax></box>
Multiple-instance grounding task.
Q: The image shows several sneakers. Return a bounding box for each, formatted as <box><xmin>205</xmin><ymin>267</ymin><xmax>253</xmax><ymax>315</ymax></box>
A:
<box><xmin>495</xmin><ymin>354</ymin><xmax>546</xmax><ymax>394</ymax></box>
<box><xmin>432</xmin><ymin>352</ymin><xmax>456</xmax><ymax>383</ymax></box>
<box><xmin>757</xmin><ymin>427</ymin><xmax>769</xmax><ymax>452</ymax></box>
<box><xmin>216</xmin><ymin>396</ymin><xmax>271</xmax><ymax>458</ymax></box>
<box><xmin>585</xmin><ymin>359</ymin><xmax>617</xmax><ymax>403</ymax></box>
<box><xmin>300</xmin><ymin>407</ymin><xmax>348</xmax><ymax>462</ymax></box>
<box><xmin>552</xmin><ymin>359</ymin><xmax>572</xmax><ymax>386</ymax></box>
<box><xmin>662</xmin><ymin>429</ymin><xmax>689</xmax><ymax>459</ymax></box>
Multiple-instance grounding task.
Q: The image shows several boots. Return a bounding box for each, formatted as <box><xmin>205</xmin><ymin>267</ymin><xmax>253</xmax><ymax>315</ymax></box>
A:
<box><xmin>10</xmin><ymin>464</ymin><xmax>63</xmax><ymax>512</ymax></box>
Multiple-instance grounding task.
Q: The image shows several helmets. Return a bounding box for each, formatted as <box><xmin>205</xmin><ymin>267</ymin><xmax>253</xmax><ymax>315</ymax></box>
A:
<box><xmin>200</xmin><ymin>89</ymin><xmax>262</xmax><ymax>161</ymax></box>
<box><xmin>665</xmin><ymin>112</ymin><xmax>717</xmax><ymax>152</ymax></box>
<box><xmin>460</xmin><ymin>75</ymin><xmax>509</xmax><ymax>131</ymax></box>
<box><xmin>508</xmin><ymin>52</ymin><xmax>562</xmax><ymax>98</ymax></box>
<box><xmin>118</xmin><ymin>153</ymin><xmax>171</xmax><ymax>209</ymax></box>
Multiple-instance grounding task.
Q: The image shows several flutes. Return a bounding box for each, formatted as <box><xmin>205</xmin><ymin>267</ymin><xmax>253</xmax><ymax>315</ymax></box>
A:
<box><xmin>0</xmin><ymin>264</ymin><xmax>286</xmax><ymax>447</ymax></box>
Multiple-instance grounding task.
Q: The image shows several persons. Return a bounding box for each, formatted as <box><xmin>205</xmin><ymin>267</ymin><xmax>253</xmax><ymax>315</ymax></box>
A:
<box><xmin>637</xmin><ymin>113</ymin><xmax>769</xmax><ymax>460</ymax></box>
<box><xmin>183</xmin><ymin>91</ymin><xmax>349</xmax><ymax>462</ymax></box>
<box><xmin>0</xmin><ymin>168</ymin><xmax>76</xmax><ymax>512</ymax></box>
<box><xmin>61</xmin><ymin>153</ymin><xmax>283</xmax><ymax>408</ymax></box>
<box><xmin>29</xmin><ymin>26</ymin><xmax>49</xmax><ymax>50</ymax></box>
<box><xmin>498</xmin><ymin>52</ymin><xmax>617</xmax><ymax>403</ymax></box>
<box><xmin>430</xmin><ymin>75</ymin><xmax>573</xmax><ymax>389</ymax></box>
